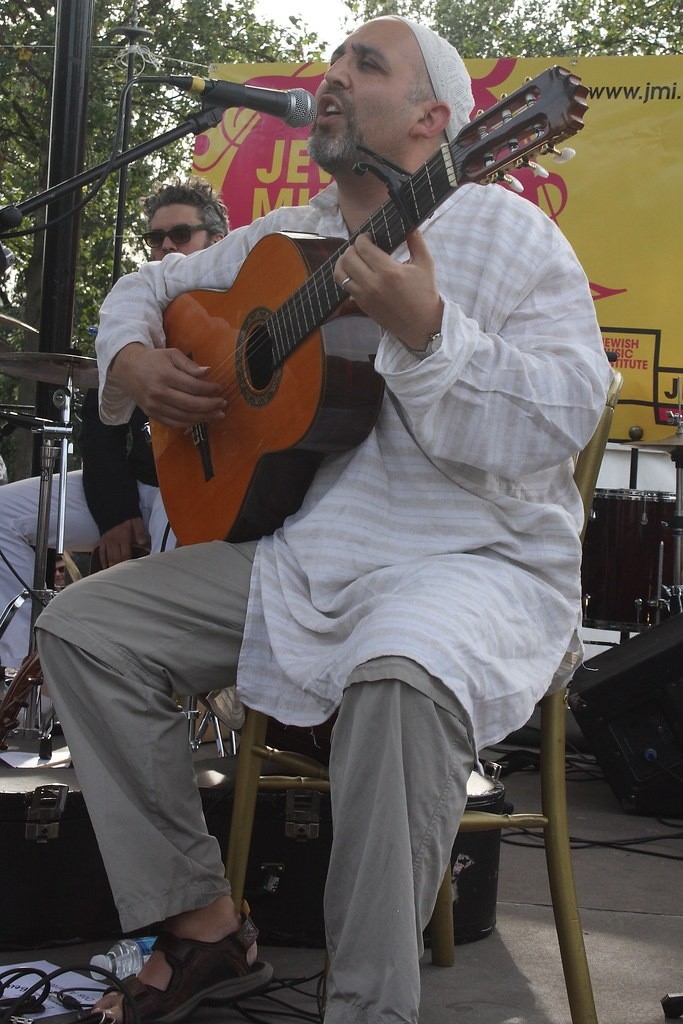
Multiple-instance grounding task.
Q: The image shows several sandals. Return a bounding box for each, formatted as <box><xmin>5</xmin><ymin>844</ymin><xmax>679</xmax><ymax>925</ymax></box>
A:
<box><xmin>93</xmin><ymin>899</ymin><xmax>273</xmax><ymax>1024</ymax></box>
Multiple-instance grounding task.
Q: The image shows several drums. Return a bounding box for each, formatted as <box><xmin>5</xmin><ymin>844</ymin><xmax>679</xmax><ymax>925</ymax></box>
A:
<box><xmin>580</xmin><ymin>487</ymin><xmax>677</xmax><ymax>632</ymax></box>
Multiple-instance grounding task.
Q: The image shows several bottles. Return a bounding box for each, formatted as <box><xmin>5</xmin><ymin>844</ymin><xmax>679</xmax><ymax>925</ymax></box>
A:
<box><xmin>89</xmin><ymin>935</ymin><xmax>158</xmax><ymax>984</ymax></box>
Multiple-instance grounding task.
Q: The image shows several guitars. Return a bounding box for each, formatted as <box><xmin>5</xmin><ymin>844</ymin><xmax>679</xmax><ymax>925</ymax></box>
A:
<box><xmin>150</xmin><ymin>65</ymin><xmax>587</xmax><ymax>546</ymax></box>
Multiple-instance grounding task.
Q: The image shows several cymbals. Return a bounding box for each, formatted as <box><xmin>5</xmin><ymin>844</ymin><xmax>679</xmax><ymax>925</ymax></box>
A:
<box><xmin>620</xmin><ymin>433</ymin><xmax>683</xmax><ymax>456</ymax></box>
<box><xmin>0</xmin><ymin>313</ymin><xmax>39</xmax><ymax>335</ymax></box>
<box><xmin>0</xmin><ymin>353</ymin><xmax>102</xmax><ymax>391</ymax></box>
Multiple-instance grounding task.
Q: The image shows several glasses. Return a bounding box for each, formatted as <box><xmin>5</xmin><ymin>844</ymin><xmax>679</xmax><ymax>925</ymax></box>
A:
<box><xmin>143</xmin><ymin>223</ymin><xmax>206</xmax><ymax>248</ymax></box>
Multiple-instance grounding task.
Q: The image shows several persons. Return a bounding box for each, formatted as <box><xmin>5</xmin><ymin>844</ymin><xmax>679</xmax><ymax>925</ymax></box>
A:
<box><xmin>0</xmin><ymin>178</ymin><xmax>231</xmax><ymax>734</ymax></box>
<box><xmin>32</xmin><ymin>13</ymin><xmax>612</xmax><ymax>1023</ymax></box>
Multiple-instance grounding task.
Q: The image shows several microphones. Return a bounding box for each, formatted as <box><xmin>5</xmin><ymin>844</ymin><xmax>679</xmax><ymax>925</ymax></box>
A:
<box><xmin>169</xmin><ymin>73</ymin><xmax>321</xmax><ymax>132</ymax></box>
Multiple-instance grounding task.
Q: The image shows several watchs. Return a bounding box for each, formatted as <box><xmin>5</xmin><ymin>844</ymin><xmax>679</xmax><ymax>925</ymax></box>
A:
<box><xmin>399</xmin><ymin>332</ymin><xmax>442</xmax><ymax>361</ymax></box>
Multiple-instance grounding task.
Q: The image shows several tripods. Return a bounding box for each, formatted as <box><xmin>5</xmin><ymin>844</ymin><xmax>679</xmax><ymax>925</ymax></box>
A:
<box><xmin>0</xmin><ymin>376</ymin><xmax>83</xmax><ymax>758</ymax></box>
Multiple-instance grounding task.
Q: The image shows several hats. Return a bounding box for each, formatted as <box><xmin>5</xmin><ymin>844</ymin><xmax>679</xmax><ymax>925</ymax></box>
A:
<box><xmin>389</xmin><ymin>14</ymin><xmax>476</xmax><ymax>144</ymax></box>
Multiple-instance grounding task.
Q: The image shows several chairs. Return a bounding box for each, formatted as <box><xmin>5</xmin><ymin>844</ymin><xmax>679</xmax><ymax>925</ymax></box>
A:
<box><xmin>225</xmin><ymin>366</ymin><xmax>625</xmax><ymax>1024</ymax></box>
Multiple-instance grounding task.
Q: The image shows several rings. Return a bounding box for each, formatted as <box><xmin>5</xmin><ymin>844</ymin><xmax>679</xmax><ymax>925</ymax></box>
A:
<box><xmin>341</xmin><ymin>275</ymin><xmax>352</xmax><ymax>293</ymax></box>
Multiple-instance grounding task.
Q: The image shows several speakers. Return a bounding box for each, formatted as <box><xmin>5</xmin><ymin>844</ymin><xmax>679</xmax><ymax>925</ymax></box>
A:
<box><xmin>567</xmin><ymin>609</ymin><xmax>683</xmax><ymax>816</ymax></box>
